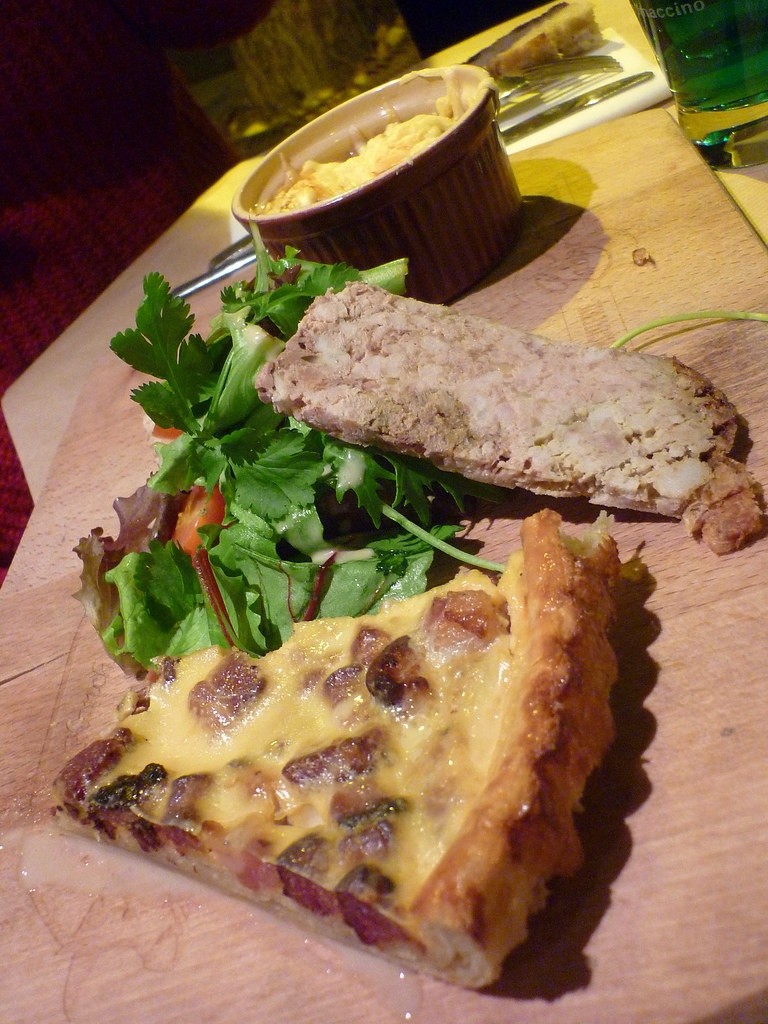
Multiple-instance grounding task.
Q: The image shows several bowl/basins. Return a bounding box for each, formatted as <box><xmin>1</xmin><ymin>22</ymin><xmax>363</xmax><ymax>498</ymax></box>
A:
<box><xmin>231</xmin><ymin>66</ymin><xmax>524</xmax><ymax>305</ymax></box>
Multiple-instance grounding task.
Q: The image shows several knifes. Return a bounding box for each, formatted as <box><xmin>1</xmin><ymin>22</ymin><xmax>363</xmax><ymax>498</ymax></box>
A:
<box><xmin>162</xmin><ymin>70</ymin><xmax>652</xmax><ymax>295</ymax></box>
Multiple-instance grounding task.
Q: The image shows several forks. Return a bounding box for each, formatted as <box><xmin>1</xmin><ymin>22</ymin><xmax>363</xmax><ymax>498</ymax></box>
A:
<box><xmin>204</xmin><ymin>54</ymin><xmax>624</xmax><ymax>267</ymax></box>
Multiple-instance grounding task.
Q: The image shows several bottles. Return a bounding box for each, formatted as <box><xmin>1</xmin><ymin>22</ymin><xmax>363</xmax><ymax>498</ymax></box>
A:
<box><xmin>628</xmin><ymin>2</ymin><xmax>767</xmax><ymax>168</ymax></box>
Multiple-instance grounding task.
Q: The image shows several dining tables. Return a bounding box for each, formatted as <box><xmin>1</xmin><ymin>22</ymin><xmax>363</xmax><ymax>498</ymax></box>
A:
<box><xmin>0</xmin><ymin>0</ymin><xmax>768</xmax><ymax>1024</ymax></box>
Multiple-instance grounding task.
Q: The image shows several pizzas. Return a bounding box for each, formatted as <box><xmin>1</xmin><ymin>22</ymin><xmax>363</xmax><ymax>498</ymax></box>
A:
<box><xmin>52</xmin><ymin>504</ymin><xmax>618</xmax><ymax>992</ymax></box>
